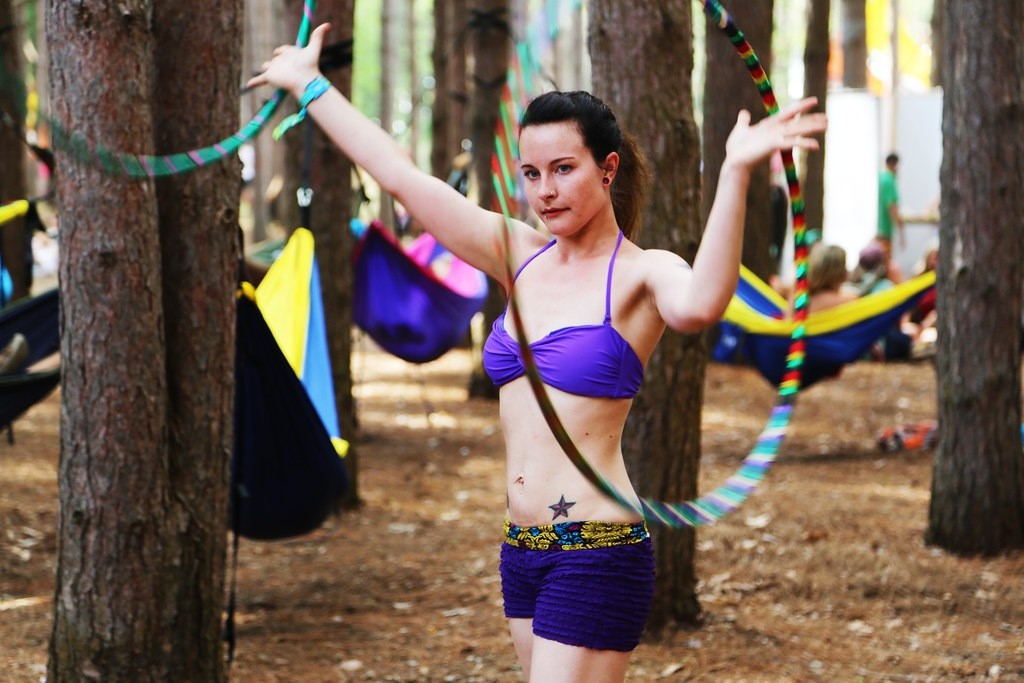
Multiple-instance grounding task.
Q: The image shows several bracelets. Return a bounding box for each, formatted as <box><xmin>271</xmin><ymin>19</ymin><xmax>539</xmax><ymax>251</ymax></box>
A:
<box><xmin>271</xmin><ymin>77</ymin><xmax>332</xmax><ymax>141</ymax></box>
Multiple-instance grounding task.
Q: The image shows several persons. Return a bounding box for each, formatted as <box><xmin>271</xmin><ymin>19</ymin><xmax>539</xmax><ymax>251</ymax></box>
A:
<box><xmin>244</xmin><ymin>22</ymin><xmax>828</xmax><ymax>683</ymax></box>
<box><xmin>805</xmin><ymin>240</ymin><xmax>938</xmax><ymax>363</ymax></box>
<box><xmin>877</xmin><ymin>154</ymin><xmax>904</xmax><ymax>238</ymax></box>
